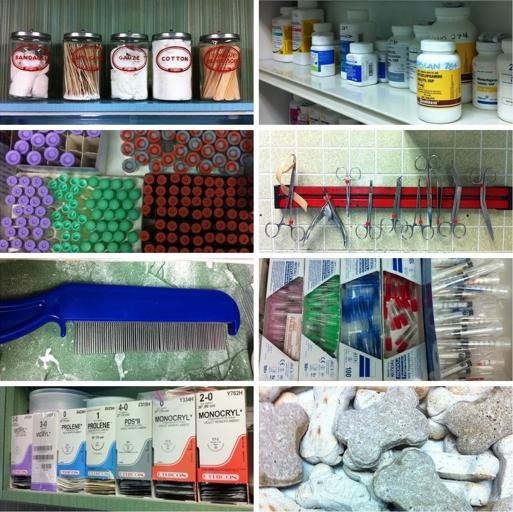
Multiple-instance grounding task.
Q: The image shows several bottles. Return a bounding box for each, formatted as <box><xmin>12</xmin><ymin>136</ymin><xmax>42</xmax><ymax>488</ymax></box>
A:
<box><xmin>269</xmin><ymin>0</ymin><xmax>513</xmax><ymax>125</ymax></box>
<box><xmin>197</xmin><ymin>32</ymin><xmax>242</xmax><ymax>103</ymax></box>
<box><xmin>108</xmin><ymin>31</ymin><xmax>150</xmax><ymax>101</ymax></box>
<box><xmin>151</xmin><ymin>31</ymin><xmax>194</xmax><ymax>101</ymax></box>
<box><xmin>62</xmin><ymin>31</ymin><xmax>103</xmax><ymax>102</ymax></box>
<box><xmin>5</xmin><ymin>30</ymin><xmax>51</xmax><ymax>101</ymax></box>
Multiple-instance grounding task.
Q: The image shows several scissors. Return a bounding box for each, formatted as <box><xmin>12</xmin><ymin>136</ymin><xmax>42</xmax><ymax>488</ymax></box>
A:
<box><xmin>264</xmin><ymin>155</ymin><xmax>498</xmax><ymax>249</ymax></box>
